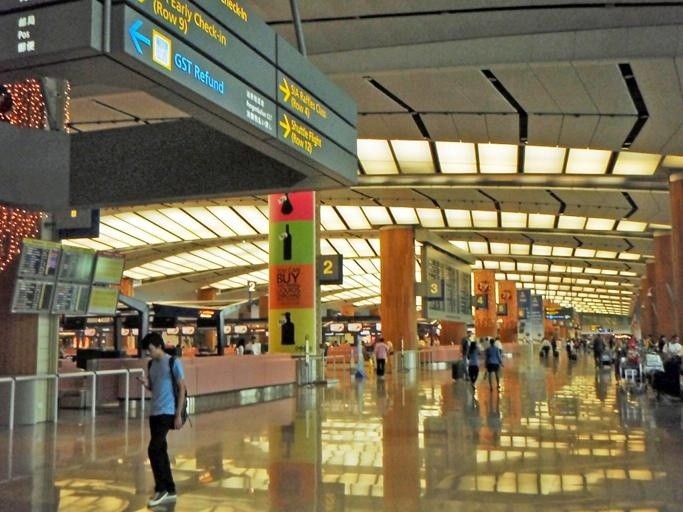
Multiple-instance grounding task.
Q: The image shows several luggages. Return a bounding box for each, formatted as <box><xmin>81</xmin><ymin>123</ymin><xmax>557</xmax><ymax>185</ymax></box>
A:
<box><xmin>451</xmin><ymin>358</ymin><xmax>466</xmax><ymax>380</ymax></box>
<box><xmin>539</xmin><ymin>350</ymin><xmax>578</xmax><ymax>360</ymax></box>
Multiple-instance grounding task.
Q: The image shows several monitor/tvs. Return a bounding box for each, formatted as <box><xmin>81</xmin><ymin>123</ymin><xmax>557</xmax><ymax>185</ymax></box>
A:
<box><xmin>84</xmin><ymin>328</ymin><xmax>96</xmax><ymax>336</ymax></box>
<box><xmin>57</xmin><ymin>245</ymin><xmax>95</xmax><ymax>284</ymax></box>
<box><xmin>348</xmin><ymin>323</ymin><xmax>362</xmax><ymax>332</ymax></box>
<box><xmin>329</xmin><ymin>323</ymin><xmax>344</xmax><ymax>332</ymax></box>
<box><xmin>86</xmin><ymin>285</ymin><xmax>120</xmax><ymax>317</ymax></box>
<box><xmin>121</xmin><ymin>325</ymin><xmax>247</xmax><ymax>336</ymax></box>
<box><xmin>51</xmin><ymin>281</ymin><xmax>90</xmax><ymax>315</ymax></box>
<box><xmin>16</xmin><ymin>238</ymin><xmax>62</xmax><ymax>281</ymax></box>
<box><xmin>11</xmin><ymin>279</ymin><xmax>55</xmax><ymax>315</ymax></box>
<box><xmin>91</xmin><ymin>253</ymin><xmax>125</xmax><ymax>285</ymax></box>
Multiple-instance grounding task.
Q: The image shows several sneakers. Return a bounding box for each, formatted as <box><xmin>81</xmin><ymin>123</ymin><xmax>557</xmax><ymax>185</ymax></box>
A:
<box><xmin>470</xmin><ymin>383</ymin><xmax>477</xmax><ymax>390</ymax></box>
<box><xmin>375</xmin><ymin>374</ymin><xmax>385</xmax><ymax>380</ymax></box>
<box><xmin>490</xmin><ymin>385</ymin><xmax>501</xmax><ymax>391</ymax></box>
<box><xmin>146</xmin><ymin>487</ymin><xmax>177</xmax><ymax>507</ymax></box>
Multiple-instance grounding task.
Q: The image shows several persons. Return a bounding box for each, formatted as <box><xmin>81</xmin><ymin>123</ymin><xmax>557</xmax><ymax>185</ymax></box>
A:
<box><xmin>461</xmin><ymin>329</ymin><xmax>503</xmax><ymax>391</ymax></box>
<box><xmin>540</xmin><ymin>335</ymin><xmax>584</xmax><ymax>362</ymax></box>
<box><xmin>235</xmin><ymin>335</ymin><xmax>261</xmax><ymax>356</ymax></box>
<box><xmin>592</xmin><ymin>333</ymin><xmax>681</xmax><ymax>367</ymax></box>
<box><xmin>374</xmin><ymin>337</ymin><xmax>389</xmax><ymax>377</ymax></box>
<box><xmin>132</xmin><ymin>331</ymin><xmax>187</xmax><ymax>508</ymax></box>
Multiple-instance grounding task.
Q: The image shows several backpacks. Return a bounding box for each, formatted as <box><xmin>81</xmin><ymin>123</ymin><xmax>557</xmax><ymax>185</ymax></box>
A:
<box><xmin>167</xmin><ymin>378</ymin><xmax>189</xmax><ymax>430</ymax></box>
